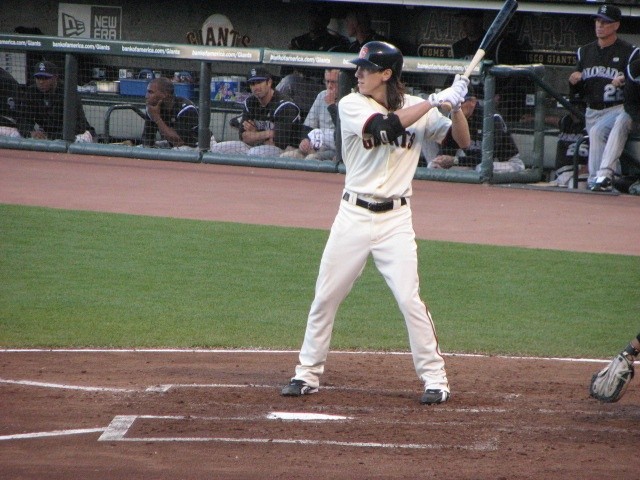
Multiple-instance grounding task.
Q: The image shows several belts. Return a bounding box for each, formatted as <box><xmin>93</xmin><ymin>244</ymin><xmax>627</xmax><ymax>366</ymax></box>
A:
<box><xmin>343</xmin><ymin>192</ymin><xmax>407</xmax><ymax>211</ymax></box>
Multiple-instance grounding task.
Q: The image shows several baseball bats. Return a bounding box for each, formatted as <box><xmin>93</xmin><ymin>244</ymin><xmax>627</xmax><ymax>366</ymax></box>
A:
<box><xmin>439</xmin><ymin>0</ymin><xmax>519</xmax><ymax>114</ymax></box>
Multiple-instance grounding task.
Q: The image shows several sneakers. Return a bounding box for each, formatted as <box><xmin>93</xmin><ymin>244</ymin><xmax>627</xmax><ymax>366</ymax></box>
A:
<box><xmin>421</xmin><ymin>389</ymin><xmax>450</xmax><ymax>403</ymax></box>
<box><xmin>591</xmin><ymin>176</ymin><xmax>613</xmax><ymax>192</ymax></box>
<box><xmin>282</xmin><ymin>380</ymin><xmax>319</xmax><ymax>396</ymax></box>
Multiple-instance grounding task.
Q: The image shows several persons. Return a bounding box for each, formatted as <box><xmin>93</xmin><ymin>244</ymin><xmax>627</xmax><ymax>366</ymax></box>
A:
<box><xmin>0</xmin><ymin>65</ymin><xmax>26</xmax><ymax>137</ymax></box>
<box><xmin>280</xmin><ymin>41</ymin><xmax>470</xmax><ymax>405</ymax></box>
<box><xmin>518</xmin><ymin>103</ymin><xmax>588</xmax><ymax>193</ymax></box>
<box><xmin>229</xmin><ymin>4</ymin><xmax>349</xmax><ymax>130</ymax></box>
<box><xmin>443</xmin><ymin>9</ymin><xmax>521</xmax><ymax>124</ymax></box>
<box><xmin>16</xmin><ymin>60</ymin><xmax>96</xmax><ymax>143</ymax></box>
<box><xmin>121</xmin><ymin>78</ymin><xmax>215</xmax><ymax>156</ymax></box>
<box><xmin>425</xmin><ymin>80</ymin><xmax>525</xmax><ymax>173</ymax></box>
<box><xmin>211</xmin><ymin>67</ymin><xmax>301</xmax><ymax>160</ymax></box>
<box><xmin>568</xmin><ymin>5</ymin><xmax>639</xmax><ymax>194</ymax></box>
<box><xmin>344</xmin><ymin>6</ymin><xmax>392</xmax><ymax>53</ymax></box>
<box><xmin>589</xmin><ymin>333</ymin><xmax>640</xmax><ymax>404</ymax></box>
<box><xmin>279</xmin><ymin>66</ymin><xmax>346</xmax><ymax>161</ymax></box>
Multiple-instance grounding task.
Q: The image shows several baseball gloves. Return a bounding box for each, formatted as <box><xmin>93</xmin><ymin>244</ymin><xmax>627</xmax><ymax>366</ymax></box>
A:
<box><xmin>589</xmin><ymin>351</ymin><xmax>634</xmax><ymax>403</ymax></box>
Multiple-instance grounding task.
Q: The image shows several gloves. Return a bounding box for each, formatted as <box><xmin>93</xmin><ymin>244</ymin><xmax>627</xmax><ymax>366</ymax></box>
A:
<box><xmin>429</xmin><ymin>74</ymin><xmax>471</xmax><ymax>108</ymax></box>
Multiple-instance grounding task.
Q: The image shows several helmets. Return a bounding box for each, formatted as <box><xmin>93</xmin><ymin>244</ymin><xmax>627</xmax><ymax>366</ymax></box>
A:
<box><xmin>350</xmin><ymin>41</ymin><xmax>403</xmax><ymax>80</ymax></box>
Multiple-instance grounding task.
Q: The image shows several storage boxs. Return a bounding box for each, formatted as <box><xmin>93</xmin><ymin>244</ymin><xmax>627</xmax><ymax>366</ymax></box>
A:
<box><xmin>118</xmin><ymin>77</ymin><xmax>150</xmax><ymax>96</ymax></box>
<box><xmin>175</xmin><ymin>81</ymin><xmax>194</xmax><ymax>99</ymax></box>
<box><xmin>210</xmin><ymin>75</ymin><xmax>248</xmax><ymax>100</ymax></box>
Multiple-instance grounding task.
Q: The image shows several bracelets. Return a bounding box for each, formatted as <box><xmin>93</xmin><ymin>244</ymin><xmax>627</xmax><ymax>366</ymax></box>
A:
<box><xmin>625</xmin><ymin>342</ymin><xmax>639</xmax><ymax>358</ymax></box>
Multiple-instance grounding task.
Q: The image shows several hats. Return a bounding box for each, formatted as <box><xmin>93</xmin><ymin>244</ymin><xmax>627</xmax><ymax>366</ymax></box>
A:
<box><xmin>33</xmin><ymin>61</ymin><xmax>59</xmax><ymax>77</ymax></box>
<box><xmin>246</xmin><ymin>67</ymin><xmax>270</xmax><ymax>82</ymax></box>
<box><xmin>590</xmin><ymin>4</ymin><xmax>622</xmax><ymax>22</ymax></box>
